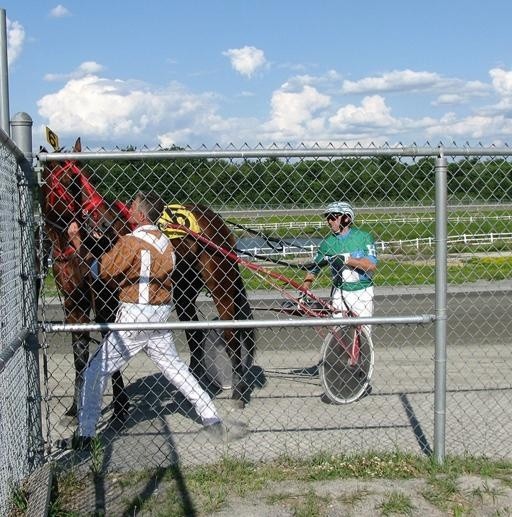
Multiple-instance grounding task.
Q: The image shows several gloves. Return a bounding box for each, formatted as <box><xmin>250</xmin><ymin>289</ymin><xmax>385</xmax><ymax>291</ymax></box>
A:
<box><xmin>322</xmin><ymin>252</ymin><xmax>351</xmax><ymax>268</ymax></box>
<box><xmin>297</xmin><ymin>289</ymin><xmax>313</xmax><ymax>312</ymax></box>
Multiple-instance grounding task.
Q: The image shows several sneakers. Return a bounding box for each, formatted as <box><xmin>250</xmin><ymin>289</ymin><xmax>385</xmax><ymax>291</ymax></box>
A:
<box><xmin>52</xmin><ymin>423</ymin><xmax>93</xmax><ymax>452</ymax></box>
<box><xmin>204</xmin><ymin>421</ymin><xmax>231</xmax><ymax>436</ymax></box>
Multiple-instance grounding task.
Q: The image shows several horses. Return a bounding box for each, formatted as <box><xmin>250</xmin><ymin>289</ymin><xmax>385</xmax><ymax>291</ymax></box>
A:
<box><xmin>38</xmin><ymin>136</ymin><xmax>257</xmax><ymax>431</ymax></box>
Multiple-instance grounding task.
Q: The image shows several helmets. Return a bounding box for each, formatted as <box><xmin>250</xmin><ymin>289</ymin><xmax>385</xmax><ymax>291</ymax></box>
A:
<box><xmin>321</xmin><ymin>201</ymin><xmax>355</xmax><ymax>225</ymax></box>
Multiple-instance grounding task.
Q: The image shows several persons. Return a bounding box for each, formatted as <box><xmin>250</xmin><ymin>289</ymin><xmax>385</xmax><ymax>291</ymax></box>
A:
<box><xmin>296</xmin><ymin>201</ymin><xmax>379</xmax><ymax>402</ymax></box>
<box><xmin>53</xmin><ymin>190</ymin><xmax>223</xmax><ymax>451</ymax></box>
